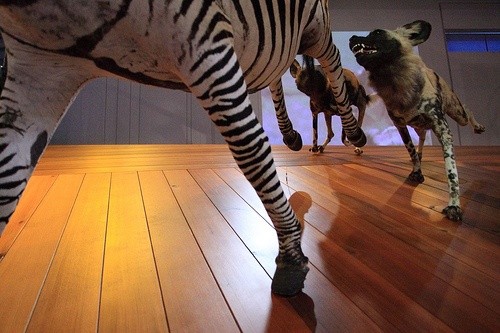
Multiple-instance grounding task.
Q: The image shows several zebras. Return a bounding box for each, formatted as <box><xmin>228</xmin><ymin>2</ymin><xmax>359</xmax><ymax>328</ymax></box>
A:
<box><xmin>0</xmin><ymin>0</ymin><xmax>367</xmax><ymax>297</ymax></box>
